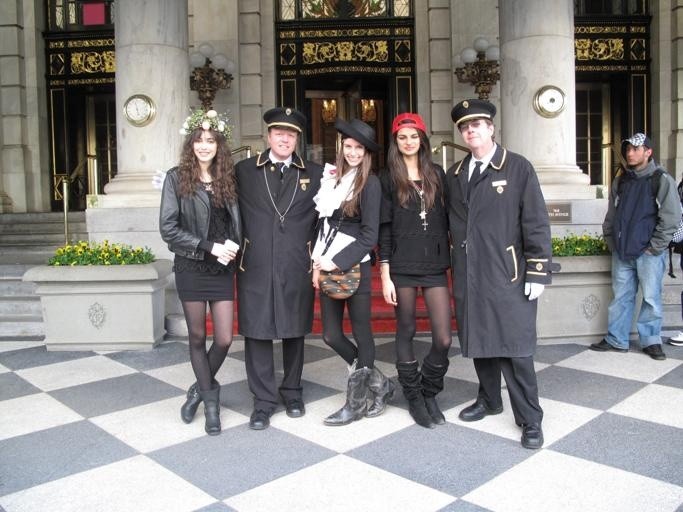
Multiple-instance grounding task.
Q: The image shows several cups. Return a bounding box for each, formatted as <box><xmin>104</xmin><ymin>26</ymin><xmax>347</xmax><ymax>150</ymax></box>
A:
<box><xmin>216</xmin><ymin>238</ymin><xmax>239</xmax><ymax>266</ymax></box>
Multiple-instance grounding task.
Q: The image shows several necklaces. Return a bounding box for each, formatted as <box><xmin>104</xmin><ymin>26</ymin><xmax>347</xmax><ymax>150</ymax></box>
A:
<box><xmin>198</xmin><ymin>179</ymin><xmax>214</xmax><ymax>188</ymax></box>
<box><xmin>417</xmin><ymin>196</ymin><xmax>430</xmax><ymax>231</ymax></box>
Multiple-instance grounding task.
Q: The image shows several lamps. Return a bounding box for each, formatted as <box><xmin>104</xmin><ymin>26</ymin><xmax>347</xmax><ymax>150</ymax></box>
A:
<box><xmin>452</xmin><ymin>37</ymin><xmax>500</xmax><ymax>104</ymax></box>
<box><xmin>187</xmin><ymin>41</ymin><xmax>237</xmax><ymax>111</ymax></box>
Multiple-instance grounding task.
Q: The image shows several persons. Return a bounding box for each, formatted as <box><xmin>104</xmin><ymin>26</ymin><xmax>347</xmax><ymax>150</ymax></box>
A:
<box><xmin>375</xmin><ymin>111</ymin><xmax>459</xmax><ymax>430</ymax></box>
<box><xmin>438</xmin><ymin>98</ymin><xmax>562</xmax><ymax>451</ymax></box>
<box><xmin>156</xmin><ymin>108</ymin><xmax>243</xmax><ymax>436</ymax></box>
<box><xmin>310</xmin><ymin>115</ymin><xmax>398</xmax><ymax>425</ymax></box>
<box><xmin>228</xmin><ymin>106</ymin><xmax>324</xmax><ymax>432</ymax></box>
<box><xmin>663</xmin><ymin>178</ymin><xmax>682</xmax><ymax>348</ymax></box>
<box><xmin>589</xmin><ymin>132</ymin><xmax>682</xmax><ymax>362</ymax></box>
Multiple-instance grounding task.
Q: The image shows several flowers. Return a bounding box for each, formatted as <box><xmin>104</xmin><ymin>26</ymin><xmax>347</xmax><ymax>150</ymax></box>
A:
<box><xmin>43</xmin><ymin>237</ymin><xmax>155</xmax><ymax>268</ymax></box>
<box><xmin>175</xmin><ymin>105</ymin><xmax>235</xmax><ymax>141</ymax></box>
<box><xmin>549</xmin><ymin>227</ymin><xmax>611</xmax><ymax>255</ymax></box>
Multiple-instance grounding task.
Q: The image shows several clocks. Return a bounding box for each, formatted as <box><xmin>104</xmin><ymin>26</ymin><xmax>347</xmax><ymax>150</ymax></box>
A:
<box><xmin>121</xmin><ymin>94</ymin><xmax>157</xmax><ymax>127</ymax></box>
<box><xmin>531</xmin><ymin>85</ymin><xmax>566</xmax><ymax>119</ymax></box>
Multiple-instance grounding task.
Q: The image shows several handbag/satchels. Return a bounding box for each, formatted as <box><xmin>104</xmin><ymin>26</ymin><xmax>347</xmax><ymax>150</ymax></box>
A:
<box><xmin>318</xmin><ymin>263</ymin><xmax>361</xmax><ymax>300</ymax></box>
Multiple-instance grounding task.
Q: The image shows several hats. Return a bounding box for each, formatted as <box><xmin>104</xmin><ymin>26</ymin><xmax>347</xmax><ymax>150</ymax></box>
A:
<box><xmin>263</xmin><ymin>107</ymin><xmax>306</xmax><ymax>135</ymax></box>
<box><xmin>622</xmin><ymin>133</ymin><xmax>652</xmax><ymax>148</ymax></box>
<box><xmin>335</xmin><ymin>116</ymin><xmax>381</xmax><ymax>152</ymax></box>
<box><xmin>392</xmin><ymin>113</ymin><xmax>425</xmax><ymax>132</ymax></box>
<box><xmin>451</xmin><ymin>98</ymin><xmax>496</xmax><ymax>128</ymax></box>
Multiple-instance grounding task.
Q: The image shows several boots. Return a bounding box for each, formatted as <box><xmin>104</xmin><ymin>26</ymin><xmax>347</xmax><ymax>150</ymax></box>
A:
<box><xmin>199</xmin><ymin>384</ymin><xmax>221</xmax><ymax>435</ymax></box>
<box><xmin>396</xmin><ymin>360</ymin><xmax>434</xmax><ymax>429</ymax></box>
<box><xmin>322</xmin><ymin>357</ymin><xmax>372</xmax><ymax>426</ymax></box>
<box><xmin>367</xmin><ymin>366</ymin><xmax>394</xmax><ymax>418</ymax></box>
<box><xmin>420</xmin><ymin>358</ymin><xmax>449</xmax><ymax>424</ymax></box>
<box><xmin>181</xmin><ymin>382</ymin><xmax>201</xmax><ymax>424</ymax></box>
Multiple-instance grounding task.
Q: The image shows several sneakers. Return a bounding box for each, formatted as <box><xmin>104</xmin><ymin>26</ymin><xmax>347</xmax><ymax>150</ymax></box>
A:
<box><xmin>668</xmin><ymin>332</ymin><xmax>683</xmax><ymax>347</ymax></box>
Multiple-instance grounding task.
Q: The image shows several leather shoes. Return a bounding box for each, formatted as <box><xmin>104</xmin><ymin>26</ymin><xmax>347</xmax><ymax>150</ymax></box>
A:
<box><xmin>286</xmin><ymin>398</ymin><xmax>304</xmax><ymax>417</ymax></box>
<box><xmin>643</xmin><ymin>344</ymin><xmax>665</xmax><ymax>359</ymax></box>
<box><xmin>249</xmin><ymin>407</ymin><xmax>273</xmax><ymax>429</ymax></box>
<box><xmin>591</xmin><ymin>339</ymin><xmax>628</xmax><ymax>352</ymax></box>
<box><xmin>520</xmin><ymin>421</ymin><xmax>543</xmax><ymax>449</ymax></box>
<box><xmin>459</xmin><ymin>401</ymin><xmax>502</xmax><ymax>421</ymax></box>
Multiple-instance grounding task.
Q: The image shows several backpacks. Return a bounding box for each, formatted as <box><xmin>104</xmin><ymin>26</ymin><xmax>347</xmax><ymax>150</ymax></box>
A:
<box><xmin>619</xmin><ymin>170</ymin><xmax>682</xmax><ymax>245</ymax></box>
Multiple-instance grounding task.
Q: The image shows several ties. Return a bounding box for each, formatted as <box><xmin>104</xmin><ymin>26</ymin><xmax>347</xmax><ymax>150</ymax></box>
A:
<box><xmin>271</xmin><ymin>163</ymin><xmax>284</xmax><ymax>195</ymax></box>
<box><xmin>469</xmin><ymin>162</ymin><xmax>482</xmax><ymax>188</ymax></box>
